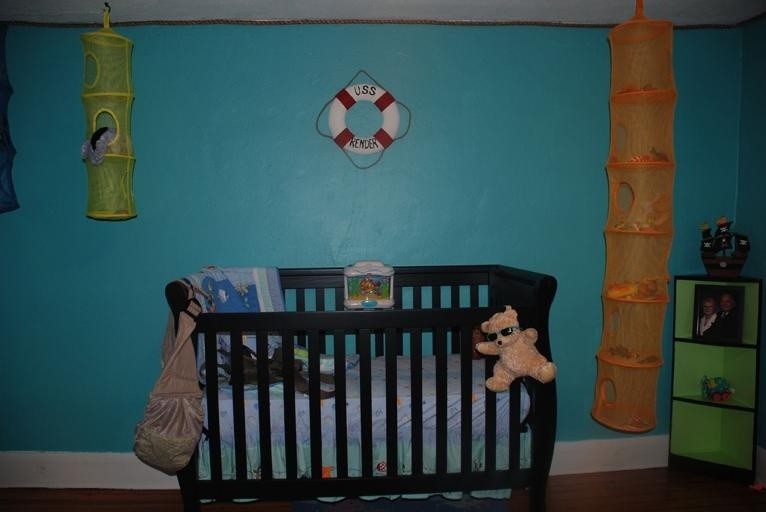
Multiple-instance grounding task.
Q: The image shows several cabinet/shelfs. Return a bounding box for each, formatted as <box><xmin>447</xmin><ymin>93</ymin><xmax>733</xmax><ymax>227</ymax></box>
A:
<box><xmin>668</xmin><ymin>273</ymin><xmax>763</xmax><ymax>484</ymax></box>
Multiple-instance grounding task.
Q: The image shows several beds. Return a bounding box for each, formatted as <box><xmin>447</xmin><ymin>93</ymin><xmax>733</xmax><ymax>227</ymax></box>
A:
<box><xmin>163</xmin><ymin>264</ymin><xmax>558</xmax><ymax>512</ymax></box>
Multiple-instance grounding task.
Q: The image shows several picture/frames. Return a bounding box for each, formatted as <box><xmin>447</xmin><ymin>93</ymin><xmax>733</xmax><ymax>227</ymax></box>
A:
<box><xmin>690</xmin><ymin>282</ymin><xmax>745</xmax><ymax>345</ymax></box>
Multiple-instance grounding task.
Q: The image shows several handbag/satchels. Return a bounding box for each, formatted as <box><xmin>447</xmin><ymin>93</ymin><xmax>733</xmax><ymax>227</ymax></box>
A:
<box><xmin>130</xmin><ymin>277</ymin><xmax>203</xmax><ymax>478</ymax></box>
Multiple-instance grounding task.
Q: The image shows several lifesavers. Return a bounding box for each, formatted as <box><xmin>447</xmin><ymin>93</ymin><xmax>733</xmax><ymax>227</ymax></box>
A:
<box><xmin>328</xmin><ymin>84</ymin><xmax>399</xmax><ymax>156</ymax></box>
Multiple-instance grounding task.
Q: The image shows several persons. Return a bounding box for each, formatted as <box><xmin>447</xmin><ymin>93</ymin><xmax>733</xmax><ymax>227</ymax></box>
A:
<box><xmin>718</xmin><ymin>293</ymin><xmax>738</xmax><ymax>341</ymax></box>
<box><xmin>695</xmin><ymin>296</ymin><xmax>721</xmax><ymax>340</ymax></box>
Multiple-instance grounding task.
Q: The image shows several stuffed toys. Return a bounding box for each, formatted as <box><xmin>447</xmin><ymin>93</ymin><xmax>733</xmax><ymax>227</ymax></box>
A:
<box><xmin>474</xmin><ymin>306</ymin><xmax>558</xmax><ymax>393</ymax></box>
